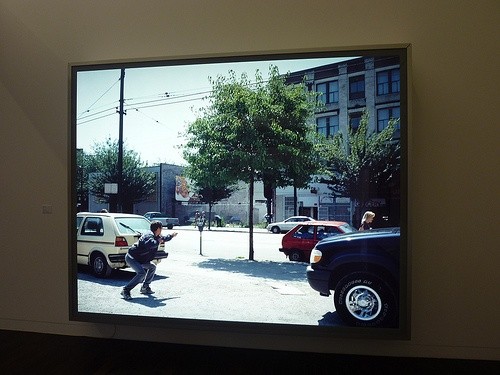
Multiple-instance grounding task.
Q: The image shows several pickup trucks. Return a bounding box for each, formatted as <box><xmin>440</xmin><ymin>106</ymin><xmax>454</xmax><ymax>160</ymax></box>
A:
<box><xmin>143</xmin><ymin>211</ymin><xmax>180</xmax><ymax>230</ymax></box>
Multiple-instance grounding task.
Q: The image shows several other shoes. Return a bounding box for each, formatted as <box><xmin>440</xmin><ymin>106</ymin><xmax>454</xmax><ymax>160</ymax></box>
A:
<box><xmin>140</xmin><ymin>286</ymin><xmax>155</xmax><ymax>294</ymax></box>
<box><xmin>120</xmin><ymin>289</ymin><xmax>132</xmax><ymax>300</ymax></box>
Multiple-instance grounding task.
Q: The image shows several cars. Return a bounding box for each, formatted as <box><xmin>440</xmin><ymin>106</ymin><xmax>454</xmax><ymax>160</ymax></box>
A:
<box><xmin>278</xmin><ymin>220</ymin><xmax>358</xmax><ymax>262</ymax></box>
<box><xmin>76</xmin><ymin>211</ymin><xmax>168</xmax><ymax>280</ymax></box>
<box><xmin>266</xmin><ymin>216</ymin><xmax>326</xmax><ymax>234</ymax></box>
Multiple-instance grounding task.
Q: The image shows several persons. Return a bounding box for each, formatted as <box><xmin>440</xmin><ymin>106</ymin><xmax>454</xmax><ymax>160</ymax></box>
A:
<box><xmin>121</xmin><ymin>220</ymin><xmax>179</xmax><ymax>300</ymax></box>
<box><xmin>201</xmin><ymin>211</ymin><xmax>206</xmax><ymax>225</ymax></box>
<box><xmin>194</xmin><ymin>209</ymin><xmax>200</xmax><ymax>228</ymax></box>
<box><xmin>358</xmin><ymin>211</ymin><xmax>376</xmax><ymax>231</ymax></box>
<box><xmin>96</xmin><ymin>209</ymin><xmax>108</xmax><ymax>233</ymax></box>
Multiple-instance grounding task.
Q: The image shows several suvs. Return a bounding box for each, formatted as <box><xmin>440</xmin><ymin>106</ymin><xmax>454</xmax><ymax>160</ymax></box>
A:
<box><xmin>306</xmin><ymin>228</ymin><xmax>401</xmax><ymax>328</ymax></box>
